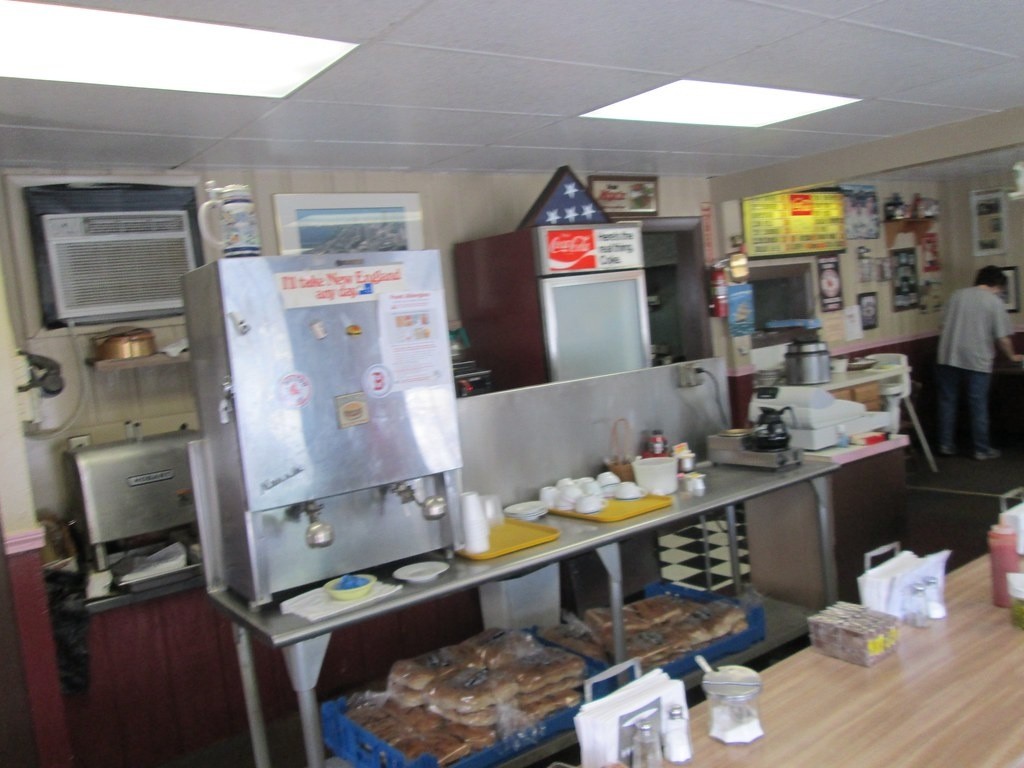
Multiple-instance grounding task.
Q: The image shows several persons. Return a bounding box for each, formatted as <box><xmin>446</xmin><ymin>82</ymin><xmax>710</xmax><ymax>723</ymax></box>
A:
<box><xmin>938</xmin><ymin>265</ymin><xmax>1024</xmax><ymax>459</ymax></box>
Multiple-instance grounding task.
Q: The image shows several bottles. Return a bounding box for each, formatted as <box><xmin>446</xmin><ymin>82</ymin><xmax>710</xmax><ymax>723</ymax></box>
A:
<box><xmin>632</xmin><ymin>719</ymin><xmax>662</xmax><ymax>768</ymax></box>
<box><xmin>986</xmin><ymin>512</ymin><xmax>1021</xmax><ymax>608</ymax></box>
<box><xmin>663</xmin><ymin>701</ymin><xmax>691</xmax><ymax>763</ymax></box>
<box><xmin>645</xmin><ymin>429</ymin><xmax>665</xmax><ymax>455</ymax></box>
<box><xmin>910</xmin><ymin>573</ymin><xmax>945</xmax><ymax>628</ymax></box>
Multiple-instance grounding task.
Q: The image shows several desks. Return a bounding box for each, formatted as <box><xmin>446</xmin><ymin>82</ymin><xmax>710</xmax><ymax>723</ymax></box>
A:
<box><xmin>603</xmin><ymin>552</ymin><xmax>1024</xmax><ymax>767</ymax></box>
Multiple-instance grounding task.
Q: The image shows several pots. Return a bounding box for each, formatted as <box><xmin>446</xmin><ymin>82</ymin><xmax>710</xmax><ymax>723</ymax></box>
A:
<box><xmin>784</xmin><ymin>335</ymin><xmax>831</xmax><ymax>385</ymax></box>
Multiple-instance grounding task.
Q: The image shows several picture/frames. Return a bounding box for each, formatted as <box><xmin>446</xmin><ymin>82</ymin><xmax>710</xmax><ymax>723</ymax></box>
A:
<box><xmin>997</xmin><ymin>266</ymin><xmax>1019</xmax><ymax>312</ymax></box>
<box><xmin>588</xmin><ymin>175</ymin><xmax>657</xmax><ymax>217</ymax></box>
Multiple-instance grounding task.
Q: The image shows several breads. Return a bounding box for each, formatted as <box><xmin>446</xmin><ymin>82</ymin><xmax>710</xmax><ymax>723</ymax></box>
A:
<box><xmin>533</xmin><ymin>593</ymin><xmax>750</xmax><ymax>674</ymax></box>
<box><xmin>343</xmin><ymin>626</ymin><xmax>587</xmax><ymax>768</ymax></box>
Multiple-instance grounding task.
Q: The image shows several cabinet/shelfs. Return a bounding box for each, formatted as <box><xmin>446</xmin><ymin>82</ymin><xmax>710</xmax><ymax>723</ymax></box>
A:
<box><xmin>62</xmin><ymin>583</ymin><xmax>482</xmax><ymax>768</ymax></box>
<box><xmin>210</xmin><ymin>435</ymin><xmax>908</xmax><ymax>768</ymax></box>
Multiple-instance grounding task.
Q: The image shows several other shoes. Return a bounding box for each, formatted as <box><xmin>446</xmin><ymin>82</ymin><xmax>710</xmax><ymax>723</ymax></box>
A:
<box><xmin>939</xmin><ymin>444</ymin><xmax>956</xmax><ymax>454</ymax></box>
<box><xmin>976</xmin><ymin>447</ymin><xmax>1002</xmax><ymax>460</ymax></box>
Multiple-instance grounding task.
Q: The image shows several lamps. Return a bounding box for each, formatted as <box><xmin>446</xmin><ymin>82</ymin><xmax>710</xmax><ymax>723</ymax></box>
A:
<box><xmin>726</xmin><ymin>250</ymin><xmax>749</xmax><ymax>280</ymax></box>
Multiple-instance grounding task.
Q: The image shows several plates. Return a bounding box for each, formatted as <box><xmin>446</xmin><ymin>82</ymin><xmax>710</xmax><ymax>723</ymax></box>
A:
<box><xmin>504</xmin><ymin>499</ymin><xmax>549</xmax><ymax>522</ymax></box>
<box><xmin>392</xmin><ymin>561</ymin><xmax>450</xmax><ymax>583</ymax></box>
<box><xmin>849</xmin><ymin>357</ymin><xmax>879</xmax><ymax>370</ymax></box>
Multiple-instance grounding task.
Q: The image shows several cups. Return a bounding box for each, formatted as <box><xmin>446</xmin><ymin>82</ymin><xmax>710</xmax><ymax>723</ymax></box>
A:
<box><xmin>537</xmin><ymin>471</ymin><xmax>648</xmax><ymax>514</ymax></box>
<box><xmin>460</xmin><ymin>490</ymin><xmax>490</xmax><ymax>555</ymax></box>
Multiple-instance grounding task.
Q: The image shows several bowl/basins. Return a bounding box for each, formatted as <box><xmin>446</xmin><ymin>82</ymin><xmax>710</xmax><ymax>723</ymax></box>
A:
<box><xmin>323</xmin><ymin>574</ymin><xmax>378</xmax><ymax>601</ymax></box>
<box><xmin>629</xmin><ymin>456</ymin><xmax>680</xmax><ymax>496</ymax></box>
<box><xmin>754</xmin><ymin>365</ymin><xmax>784</xmax><ymax>385</ymax></box>
<box><xmin>97</xmin><ymin>333</ymin><xmax>155</xmax><ymax>357</ymax></box>
<box><xmin>831</xmin><ymin>359</ymin><xmax>848</xmax><ymax>373</ymax></box>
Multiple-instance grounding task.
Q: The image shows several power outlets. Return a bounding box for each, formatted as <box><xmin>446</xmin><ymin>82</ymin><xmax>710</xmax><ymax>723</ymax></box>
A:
<box><xmin>685</xmin><ymin>360</ymin><xmax>704</xmax><ymax>386</ymax></box>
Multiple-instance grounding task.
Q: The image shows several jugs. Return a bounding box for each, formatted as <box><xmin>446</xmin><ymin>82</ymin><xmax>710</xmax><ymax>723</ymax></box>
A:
<box><xmin>749</xmin><ymin>405</ymin><xmax>797</xmax><ymax>451</ymax></box>
<box><xmin>198</xmin><ymin>177</ymin><xmax>263</xmax><ymax>258</ymax></box>
